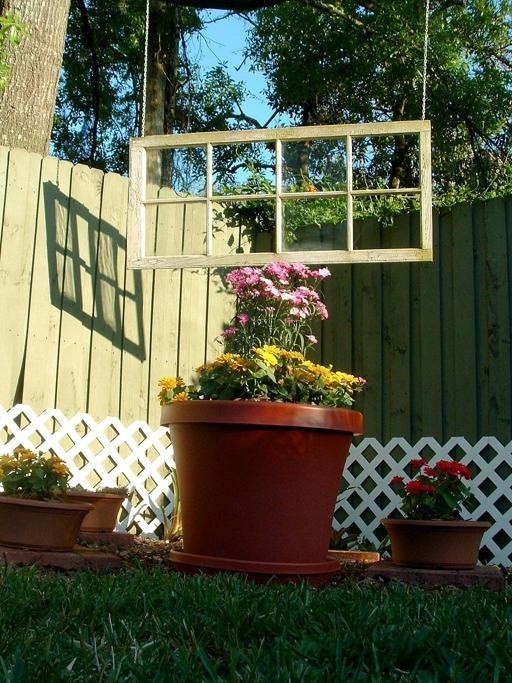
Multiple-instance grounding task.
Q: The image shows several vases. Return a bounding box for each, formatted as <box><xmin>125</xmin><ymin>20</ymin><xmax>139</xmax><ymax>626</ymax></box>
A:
<box><xmin>0</xmin><ymin>495</ymin><xmax>96</xmax><ymax>553</ymax></box>
<box><xmin>70</xmin><ymin>492</ymin><xmax>127</xmax><ymax>533</ymax></box>
<box><xmin>389</xmin><ymin>456</ymin><xmax>473</xmax><ymax>517</ymax></box>
<box><xmin>160</xmin><ymin>397</ymin><xmax>364</xmax><ymax>590</ymax></box>
<box><xmin>379</xmin><ymin>517</ymin><xmax>492</xmax><ymax>568</ymax></box>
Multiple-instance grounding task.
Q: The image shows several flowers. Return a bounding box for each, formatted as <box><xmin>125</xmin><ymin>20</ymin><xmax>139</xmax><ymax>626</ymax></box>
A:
<box><xmin>156</xmin><ymin>259</ymin><xmax>369</xmax><ymax>408</ymax></box>
<box><xmin>0</xmin><ymin>444</ymin><xmax>72</xmax><ymax>504</ymax></box>
